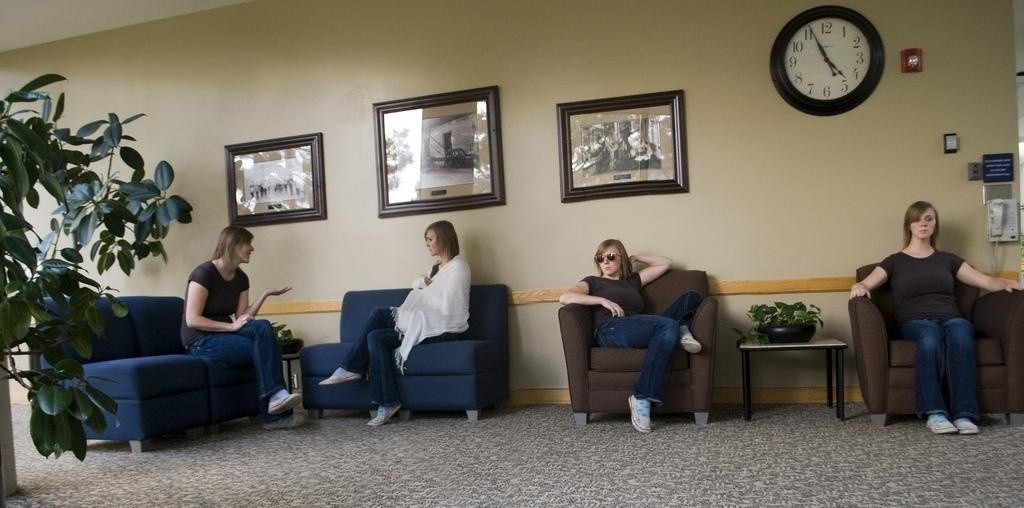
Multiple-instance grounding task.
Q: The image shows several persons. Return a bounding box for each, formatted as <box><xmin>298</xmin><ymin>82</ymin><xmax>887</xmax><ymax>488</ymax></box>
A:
<box><xmin>557</xmin><ymin>238</ymin><xmax>706</xmax><ymax>434</ymax></box>
<box><xmin>179</xmin><ymin>225</ymin><xmax>311</xmax><ymax>431</ymax></box>
<box><xmin>248</xmin><ymin>176</ymin><xmax>299</xmax><ymax>200</ymax></box>
<box><xmin>583</xmin><ymin>126</ymin><xmax>661</xmax><ymax>175</ymax></box>
<box><xmin>315</xmin><ymin>220</ymin><xmax>471</xmax><ymax>429</ymax></box>
<box><xmin>847</xmin><ymin>199</ymin><xmax>1024</xmax><ymax>436</ymax></box>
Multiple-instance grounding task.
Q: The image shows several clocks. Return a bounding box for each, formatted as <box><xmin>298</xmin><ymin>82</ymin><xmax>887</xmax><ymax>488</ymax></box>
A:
<box><xmin>769</xmin><ymin>5</ymin><xmax>885</xmax><ymax>118</ymax></box>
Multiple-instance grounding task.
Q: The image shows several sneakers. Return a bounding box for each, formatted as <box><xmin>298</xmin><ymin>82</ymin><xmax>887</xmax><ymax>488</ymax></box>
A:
<box><xmin>262</xmin><ymin>410</ymin><xmax>308</xmax><ymax>431</ymax></box>
<box><xmin>628</xmin><ymin>392</ymin><xmax>654</xmax><ymax>434</ymax></box>
<box><xmin>925</xmin><ymin>412</ymin><xmax>959</xmax><ymax>436</ymax></box>
<box><xmin>954</xmin><ymin>418</ymin><xmax>981</xmax><ymax>435</ymax></box>
<box><xmin>366</xmin><ymin>400</ymin><xmax>403</xmax><ymax>427</ymax></box>
<box><xmin>267</xmin><ymin>389</ymin><xmax>303</xmax><ymax>415</ymax></box>
<box><xmin>318</xmin><ymin>366</ymin><xmax>363</xmax><ymax>386</ymax></box>
<box><xmin>678</xmin><ymin>325</ymin><xmax>703</xmax><ymax>354</ymax></box>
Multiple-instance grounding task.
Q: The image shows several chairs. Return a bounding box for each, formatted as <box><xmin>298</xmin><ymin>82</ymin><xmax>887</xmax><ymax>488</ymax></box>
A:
<box><xmin>555</xmin><ymin>267</ymin><xmax>719</xmax><ymax>427</ymax></box>
<box><xmin>845</xmin><ymin>260</ymin><xmax>1023</xmax><ymax>428</ymax></box>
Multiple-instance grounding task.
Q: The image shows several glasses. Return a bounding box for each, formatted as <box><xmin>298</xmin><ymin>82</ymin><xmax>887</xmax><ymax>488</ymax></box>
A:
<box><xmin>595</xmin><ymin>252</ymin><xmax>624</xmax><ymax>263</ymax></box>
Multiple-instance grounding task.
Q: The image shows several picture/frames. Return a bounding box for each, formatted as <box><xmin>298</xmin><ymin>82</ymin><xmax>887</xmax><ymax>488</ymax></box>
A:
<box><xmin>554</xmin><ymin>89</ymin><xmax>689</xmax><ymax>203</ymax></box>
<box><xmin>372</xmin><ymin>85</ymin><xmax>506</xmax><ymax>219</ymax></box>
<box><xmin>222</xmin><ymin>132</ymin><xmax>330</xmax><ymax>228</ymax></box>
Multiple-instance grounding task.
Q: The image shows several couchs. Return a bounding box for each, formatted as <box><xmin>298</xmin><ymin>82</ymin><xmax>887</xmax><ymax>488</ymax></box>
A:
<box><xmin>31</xmin><ymin>294</ymin><xmax>275</xmax><ymax>456</ymax></box>
<box><xmin>299</xmin><ymin>283</ymin><xmax>509</xmax><ymax>423</ymax></box>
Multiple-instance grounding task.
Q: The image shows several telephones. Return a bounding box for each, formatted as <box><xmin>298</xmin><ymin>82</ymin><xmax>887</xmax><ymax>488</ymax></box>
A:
<box><xmin>987</xmin><ymin>199</ymin><xmax>1019</xmax><ymax>242</ymax></box>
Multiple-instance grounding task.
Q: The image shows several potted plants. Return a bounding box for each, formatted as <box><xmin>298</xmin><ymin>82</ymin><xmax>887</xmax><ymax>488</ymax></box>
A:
<box><xmin>728</xmin><ymin>294</ymin><xmax>824</xmax><ymax>350</ymax></box>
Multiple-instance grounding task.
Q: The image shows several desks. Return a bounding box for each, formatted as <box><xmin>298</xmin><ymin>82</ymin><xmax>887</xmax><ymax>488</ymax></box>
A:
<box><xmin>270</xmin><ymin>352</ymin><xmax>300</xmax><ymax>417</ymax></box>
<box><xmin>736</xmin><ymin>333</ymin><xmax>848</xmax><ymax>422</ymax></box>
